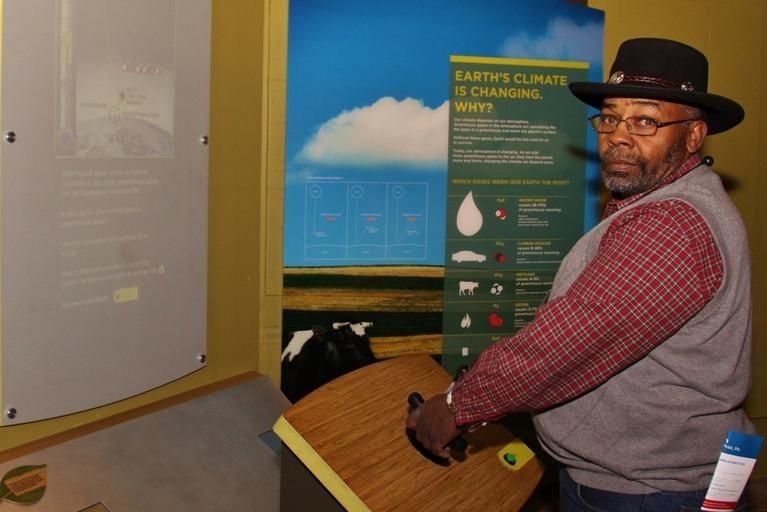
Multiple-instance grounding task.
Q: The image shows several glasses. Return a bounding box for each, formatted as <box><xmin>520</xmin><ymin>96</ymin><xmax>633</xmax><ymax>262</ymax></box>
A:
<box><xmin>588</xmin><ymin>114</ymin><xmax>700</xmax><ymax>136</ymax></box>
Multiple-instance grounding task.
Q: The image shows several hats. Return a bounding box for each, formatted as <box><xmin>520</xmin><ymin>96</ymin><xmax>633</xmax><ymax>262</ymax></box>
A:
<box><xmin>567</xmin><ymin>38</ymin><xmax>744</xmax><ymax>136</ymax></box>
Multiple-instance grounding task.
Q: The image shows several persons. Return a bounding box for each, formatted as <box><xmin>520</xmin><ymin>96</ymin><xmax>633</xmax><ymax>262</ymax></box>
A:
<box><xmin>402</xmin><ymin>30</ymin><xmax>762</xmax><ymax>511</ymax></box>
